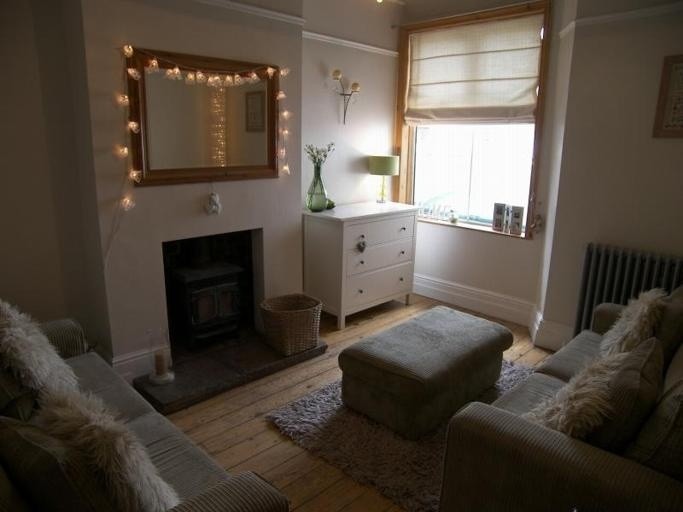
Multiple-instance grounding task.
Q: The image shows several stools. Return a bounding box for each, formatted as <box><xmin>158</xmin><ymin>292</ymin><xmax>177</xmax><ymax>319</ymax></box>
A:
<box><xmin>338</xmin><ymin>304</ymin><xmax>512</xmax><ymax>439</ymax></box>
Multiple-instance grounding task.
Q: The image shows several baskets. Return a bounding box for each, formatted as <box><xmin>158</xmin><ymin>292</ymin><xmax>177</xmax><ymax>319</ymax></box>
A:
<box><xmin>260</xmin><ymin>293</ymin><xmax>323</xmax><ymax>356</ymax></box>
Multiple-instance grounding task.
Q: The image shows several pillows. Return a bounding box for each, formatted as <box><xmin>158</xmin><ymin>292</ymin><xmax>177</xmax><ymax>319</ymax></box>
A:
<box><xmin>652</xmin><ymin>285</ymin><xmax>683</xmax><ymax>351</ymax></box>
<box><xmin>521</xmin><ymin>349</ymin><xmax>632</xmax><ymax>435</ymax></box>
<box><xmin>0</xmin><ymin>415</ymin><xmax>113</xmax><ymax>512</ymax></box>
<box><xmin>0</xmin><ymin>298</ymin><xmax>79</xmax><ymax>393</ymax></box>
<box><xmin>41</xmin><ymin>389</ymin><xmax>181</xmax><ymax>512</ymax></box>
<box><xmin>577</xmin><ymin>337</ymin><xmax>665</xmax><ymax>448</ymax></box>
<box><xmin>0</xmin><ymin>356</ymin><xmax>36</xmax><ymax>420</ymax></box>
<box><xmin>599</xmin><ymin>286</ymin><xmax>667</xmax><ymax>356</ymax></box>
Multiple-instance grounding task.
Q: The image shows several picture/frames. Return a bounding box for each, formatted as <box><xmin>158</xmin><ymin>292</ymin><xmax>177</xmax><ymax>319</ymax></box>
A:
<box><xmin>243</xmin><ymin>90</ymin><xmax>266</xmax><ymax>133</ymax></box>
<box><xmin>652</xmin><ymin>54</ymin><xmax>683</xmax><ymax>138</ymax></box>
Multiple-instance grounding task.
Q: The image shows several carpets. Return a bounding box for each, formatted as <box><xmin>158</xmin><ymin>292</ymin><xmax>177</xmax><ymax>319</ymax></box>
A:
<box><xmin>261</xmin><ymin>358</ymin><xmax>535</xmax><ymax>512</ymax></box>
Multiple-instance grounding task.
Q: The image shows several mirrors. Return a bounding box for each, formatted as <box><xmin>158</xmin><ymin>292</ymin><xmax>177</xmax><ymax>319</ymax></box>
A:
<box><xmin>127</xmin><ymin>46</ymin><xmax>279</xmax><ymax>187</ymax></box>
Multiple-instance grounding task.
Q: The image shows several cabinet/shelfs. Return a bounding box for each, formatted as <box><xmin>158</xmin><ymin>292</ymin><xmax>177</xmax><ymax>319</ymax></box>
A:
<box><xmin>303</xmin><ymin>199</ymin><xmax>419</xmax><ymax>329</ymax></box>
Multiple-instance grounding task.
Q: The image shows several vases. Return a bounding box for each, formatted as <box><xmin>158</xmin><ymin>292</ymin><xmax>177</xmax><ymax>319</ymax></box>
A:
<box><xmin>305</xmin><ymin>161</ymin><xmax>328</xmax><ymax>212</ymax></box>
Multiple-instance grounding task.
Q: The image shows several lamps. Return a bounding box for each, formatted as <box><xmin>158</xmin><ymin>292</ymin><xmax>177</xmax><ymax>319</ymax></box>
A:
<box><xmin>368</xmin><ymin>155</ymin><xmax>400</xmax><ymax>204</ymax></box>
<box><xmin>333</xmin><ymin>68</ymin><xmax>361</xmax><ymax>124</ymax></box>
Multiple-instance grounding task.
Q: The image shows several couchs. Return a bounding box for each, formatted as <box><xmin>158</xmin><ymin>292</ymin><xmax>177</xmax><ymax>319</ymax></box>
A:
<box><xmin>441</xmin><ymin>287</ymin><xmax>683</xmax><ymax>512</ymax></box>
<box><xmin>0</xmin><ymin>298</ymin><xmax>289</xmax><ymax>512</ymax></box>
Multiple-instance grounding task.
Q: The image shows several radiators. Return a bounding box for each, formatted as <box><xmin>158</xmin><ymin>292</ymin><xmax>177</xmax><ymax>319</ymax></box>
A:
<box><xmin>574</xmin><ymin>240</ymin><xmax>683</xmax><ymax>336</ymax></box>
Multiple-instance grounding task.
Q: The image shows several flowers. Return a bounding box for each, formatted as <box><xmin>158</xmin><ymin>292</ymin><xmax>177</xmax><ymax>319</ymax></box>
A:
<box><xmin>304</xmin><ymin>140</ymin><xmax>336</xmax><ymax>194</ymax></box>
